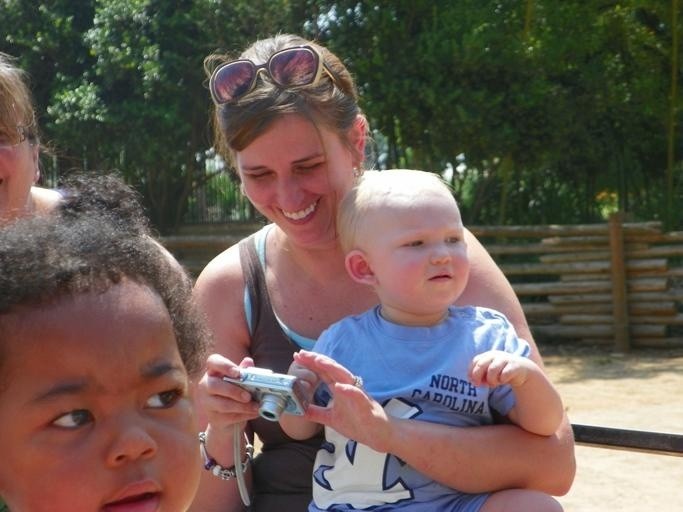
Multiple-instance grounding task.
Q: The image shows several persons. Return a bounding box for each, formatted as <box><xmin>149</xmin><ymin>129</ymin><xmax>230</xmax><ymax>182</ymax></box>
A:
<box><xmin>275</xmin><ymin>167</ymin><xmax>563</xmax><ymax>510</ymax></box>
<box><xmin>0</xmin><ymin>49</ymin><xmax>191</xmax><ymax>301</ymax></box>
<box><xmin>0</xmin><ymin>211</ymin><xmax>213</xmax><ymax>510</ymax></box>
<box><xmin>181</xmin><ymin>32</ymin><xmax>578</xmax><ymax>511</ymax></box>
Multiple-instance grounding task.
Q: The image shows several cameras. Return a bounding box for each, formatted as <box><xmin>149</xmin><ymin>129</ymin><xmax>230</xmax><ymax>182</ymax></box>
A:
<box><xmin>221</xmin><ymin>364</ymin><xmax>312</xmax><ymax>422</ymax></box>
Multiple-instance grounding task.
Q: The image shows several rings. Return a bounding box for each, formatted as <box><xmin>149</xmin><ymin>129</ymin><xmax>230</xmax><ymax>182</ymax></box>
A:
<box><xmin>352</xmin><ymin>374</ymin><xmax>364</xmax><ymax>387</ymax></box>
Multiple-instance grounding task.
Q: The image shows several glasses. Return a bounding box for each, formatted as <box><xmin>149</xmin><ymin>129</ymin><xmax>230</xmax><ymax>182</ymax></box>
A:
<box><xmin>209</xmin><ymin>44</ymin><xmax>349</xmax><ymax>105</ymax></box>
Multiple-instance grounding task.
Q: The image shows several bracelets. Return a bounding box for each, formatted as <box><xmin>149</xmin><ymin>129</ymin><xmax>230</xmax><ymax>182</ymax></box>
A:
<box><xmin>197</xmin><ymin>428</ymin><xmax>253</xmax><ymax>482</ymax></box>
